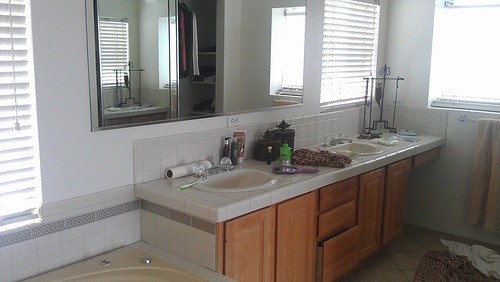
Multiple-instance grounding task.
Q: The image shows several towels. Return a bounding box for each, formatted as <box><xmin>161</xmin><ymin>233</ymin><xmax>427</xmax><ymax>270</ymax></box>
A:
<box><xmin>291</xmin><ymin>149</ymin><xmax>352</xmax><ymax>168</ymax></box>
<box><xmin>466</xmin><ymin>119</ymin><xmax>500</xmax><ymax>234</ymax></box>
<box><xmin>414</xmin><ymin>238</ymin><xmax>500</xmax><ymax>282</ymax></box>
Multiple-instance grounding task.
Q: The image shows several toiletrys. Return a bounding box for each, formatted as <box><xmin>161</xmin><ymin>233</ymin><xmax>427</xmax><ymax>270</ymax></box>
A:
<box><xmin>177</xmin><ymin>177</ymin><xmax>208</xmax><ymax>191</ymax></box>
<box><xmin>267</xmin><ymin>146</ymin><xmax>272</xmax><ymax>165</ymax></box>
<box><xmin>280</xmin><ymin>141</ymin><xmax>291</xmax><ymax>167</ymax></box>
<box><xmin>224</xmin><ymin>130</ymin><xmax>246</xmax><ymax>165</ymax></box>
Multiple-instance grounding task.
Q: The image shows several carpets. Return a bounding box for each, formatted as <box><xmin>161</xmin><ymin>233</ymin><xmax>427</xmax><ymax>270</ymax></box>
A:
<box><xmin>413</xmin><ymin>250</ymin><xmax>500</xmax><ymax>282</ymax></box>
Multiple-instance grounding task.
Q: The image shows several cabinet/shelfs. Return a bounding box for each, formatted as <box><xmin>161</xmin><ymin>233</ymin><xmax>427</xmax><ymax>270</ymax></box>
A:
<box><xmin>104</xmin><ymin>113</ymin><xmax>169</xmax><ymax>126</ymax></box>
<box><xmin>178</xmin><ymin>1</ymin><xmax>217</xmax><ymax>116</ymax></box>
<box><xmin>214</xmin><ymin>147</ymin><xmax>441</xmax><ymax>282</ymax></box>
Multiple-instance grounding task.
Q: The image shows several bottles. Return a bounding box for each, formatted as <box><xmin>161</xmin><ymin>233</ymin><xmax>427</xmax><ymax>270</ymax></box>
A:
<box><xmin>231</xmin><ymin>138</ymin><xmax>238</xmax><ymax>165</ymax></box>
<box><xmin>224</xmin><ymin>137</ymin><xmax>230</xmax><ymax>158</ymax></box>
<box><xmin>280</xmin><ymin>141</ymin><xmax>291</xmax><ymax>165</ymax></box>
<box><xmin>267</xmin><ymin>146</ymin><xmax>272</xmax><ymax>165</ymax></box>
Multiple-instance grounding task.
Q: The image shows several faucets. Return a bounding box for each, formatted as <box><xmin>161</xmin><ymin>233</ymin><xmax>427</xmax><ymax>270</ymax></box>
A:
<box><xmin>209</xmin><ymin>166</ymin><xmax>221</xmax><ymax>174</ymax></box>
<box><xmin>330</xmin><ymin>138</ymin><xmax>353</xmax><ymax>146</ymax></box>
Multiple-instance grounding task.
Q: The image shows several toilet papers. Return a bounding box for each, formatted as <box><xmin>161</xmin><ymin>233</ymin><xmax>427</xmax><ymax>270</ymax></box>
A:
<box><xmin>166</xmin><ymin>160</ymin><xmax>212</xmax><ymax>179</ymax></box>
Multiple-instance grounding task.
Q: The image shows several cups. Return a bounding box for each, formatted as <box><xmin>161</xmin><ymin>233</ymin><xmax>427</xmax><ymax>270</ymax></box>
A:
<box><xmin>193</xmin><ymin>160</ymin><xmax>205</xmax><ymax>177</ymax></box>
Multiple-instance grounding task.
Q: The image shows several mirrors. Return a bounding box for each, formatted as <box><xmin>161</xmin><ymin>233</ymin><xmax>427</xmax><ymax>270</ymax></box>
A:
<box><xmin>93</xmin><ymin>0</ymin><xmax>307</xmax><ymax>128</ymax></box>
<box><xmin>274</xmin><ymin>165</ymin><xmax>319</xmax><ymax>174</ymax></box>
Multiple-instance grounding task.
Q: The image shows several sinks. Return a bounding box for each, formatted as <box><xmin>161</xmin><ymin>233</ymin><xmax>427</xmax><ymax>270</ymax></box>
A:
<box><xmin>327</xmin><ymin>142</ymin><xmax>384</xmax><ymax>156</ymax></box>
<box><xmin>187</xmin><ymin>167</ymin><xmax>277</xmax><ymax>192</ymax></box>
